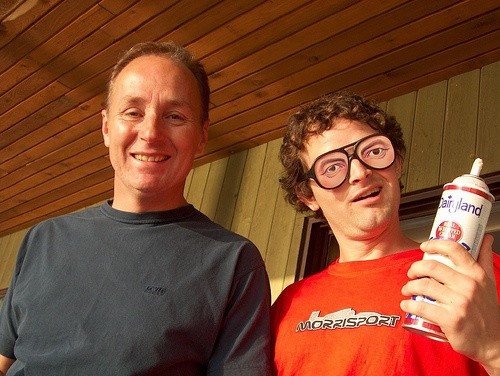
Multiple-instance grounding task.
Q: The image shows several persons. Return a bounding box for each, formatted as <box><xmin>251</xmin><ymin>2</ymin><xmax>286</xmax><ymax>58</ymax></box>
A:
<box><xmin>0</xmin><ymin>39</ymin><xmax>275</xmax><ymax>376</ymax></box>
<box><xmin>271</xmin><ymin>93</ymin><xmax>500</xmax><ymax>376</ymax></box>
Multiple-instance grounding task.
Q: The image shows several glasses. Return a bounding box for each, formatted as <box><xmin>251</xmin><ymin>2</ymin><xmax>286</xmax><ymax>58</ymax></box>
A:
<box><xmin>304</xmin><ymin>133</ymin><xmax>396</xmax><ymax>190</ymax></box>
